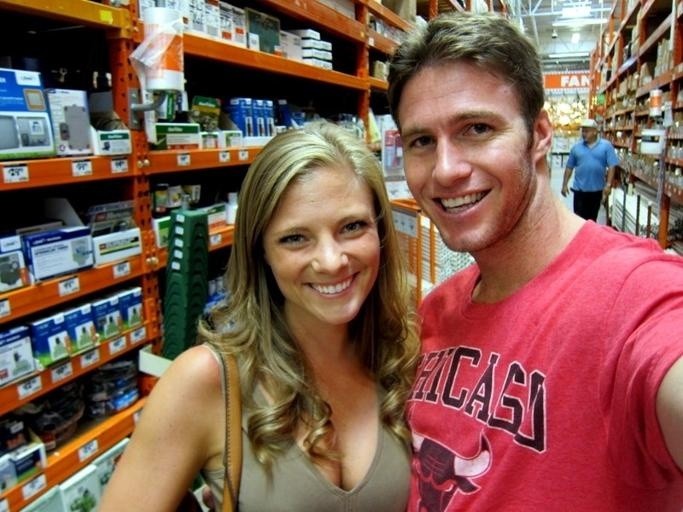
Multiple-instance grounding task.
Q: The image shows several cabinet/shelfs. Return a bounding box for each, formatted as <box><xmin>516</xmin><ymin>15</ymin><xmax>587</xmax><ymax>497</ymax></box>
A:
<box><xmin>0</xmin><ymin>0</ymin><xmax>511</xmax><ymax>511</ymax></box>
<box><xmin>582</xmin><ymin>0</ymin><xmax>682</xmax><ymax>262</ymax></box>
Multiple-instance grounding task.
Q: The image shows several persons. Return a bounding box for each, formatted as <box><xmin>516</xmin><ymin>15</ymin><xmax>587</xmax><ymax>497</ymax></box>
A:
<box><xmin>95</xmin><ymin>119</ymin><xmax>412</xmax><ymax>512</ymax></box>
<box><xmin>387</xmin><ymin>11</ymin><xmax>683</xmax><ymax>512</ymax></box>
<box><xmin>562</xmin><ymin>119</ymin><xmax>619</xmax><ymax>223</ymax></box>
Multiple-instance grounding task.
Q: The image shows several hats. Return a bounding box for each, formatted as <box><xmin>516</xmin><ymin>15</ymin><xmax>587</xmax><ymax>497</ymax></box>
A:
<box><xmin>579</xmin><ymin>118</ymin><xmax>597</xmax><ymax>128</ymax></box>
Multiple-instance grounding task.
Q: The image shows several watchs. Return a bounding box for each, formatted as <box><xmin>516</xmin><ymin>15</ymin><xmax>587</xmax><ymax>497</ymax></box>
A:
<box><xmin>607</xmin><ymin>183</ymin><xmax>613</xmax><ymax>187</ymax></box>
